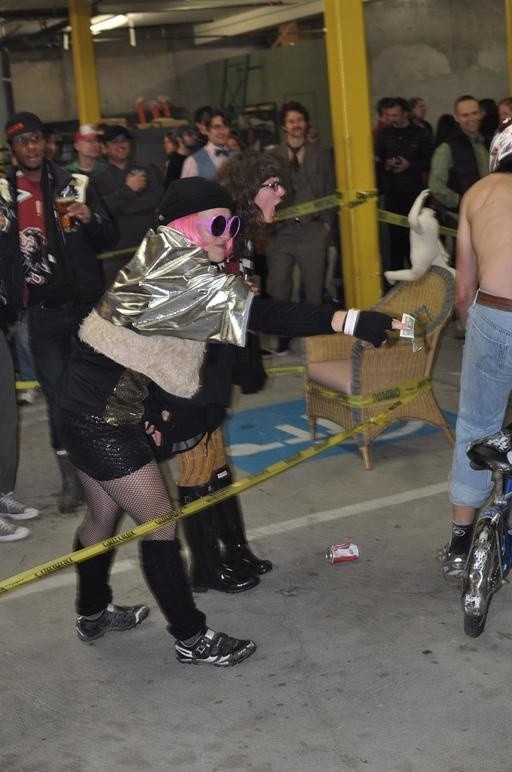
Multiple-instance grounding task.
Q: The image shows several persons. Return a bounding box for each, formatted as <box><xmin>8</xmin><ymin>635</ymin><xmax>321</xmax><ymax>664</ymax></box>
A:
<box><xmin>0</xmin><ymin>166</ymin><xmax>40</xmax><ymax>543</ymax></box>
<box><xmin>90</xmin><ymin>124</ymin><xmax>166</xmax><ymax>290</ymax></box>
<box><xmin>305</xmin><ymin>125</ymin><xmax>337</xmax><ymax>294</ymax></box>
<box><xmin>436</xmin><ymin>122</ymin><xmax>511</xmax><ymax>579</ymax></box>
<box><xmin>60</xmin><ymin>123</ymin><xmax>109</xmax><ymax>180</ymax></box>
<box><xmin>53</xmin><ymin>177</ymin><xmax>412</xmax><ymax>667</ymax></box>
<box><xmin>373</xmin><ymin>96</ymin><xmax>512</xmax><ymax>297</ymax></box>
<box><xmin>162</xmin><ymin>106</ymin><xmax>245</xmax><ymax>183</ymax></box>
<box><xmin>46</xmin><ymin>129</ymin><xmax>63</xmax><ymax>158</ymax></box>
<box><xmin>6</xmin><ymin>111</ymin><xmax>120</xmax><ymax>514</ymax></box>
<box><xmin>263</xmin><ymin>100</ymin><xmax>338</xmax><ymax>354</ymax></box>
<box><xmin>159</xmin><ymin>148</ymin><xmax>288</xmax><ymax>593</ymax></box>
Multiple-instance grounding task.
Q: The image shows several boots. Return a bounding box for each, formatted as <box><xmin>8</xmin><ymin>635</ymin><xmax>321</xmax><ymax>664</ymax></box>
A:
<box><xmin>176</xmin><ymin>480</ymin><xmax>260</xmax><ymax>593</ymax></box>
<box><xmin>210</xmin><ymin>464</ymin><xmax>272</xmax><ymax>575</ymax></box>
<box><xmin>57</xmin><ymin>452</ymin><xmax>82</xmax><ymax>514</ymax></box>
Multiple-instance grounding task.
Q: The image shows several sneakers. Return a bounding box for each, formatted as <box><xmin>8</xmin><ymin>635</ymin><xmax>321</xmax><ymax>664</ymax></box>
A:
<box><xmin>0</xmin><ymin>517</ymin><xmax>30</xmax><ymax>542</ymax></box>
<box><xmin>16</xmin><ymin>387</ymin><xmax>37</xmax><ymax>403</ymax></box>
<box><xmin>0</xmin><ymin>491</ymin><xmax>39</xmax><ymax>521</ymax></box>
<box><xmin>174</xmin><ymin>627</ymin><xmax>257</xmax><ymax>667</ymax></box>
<box><xmin>75</xmin><ymin>605</ymin><xmax>150</xmax><ymax>641</ymax></box>
<box><xmin>436</xmin><ymin>543</ymin><xmax>469</xmax><ymax>590</ymax></box>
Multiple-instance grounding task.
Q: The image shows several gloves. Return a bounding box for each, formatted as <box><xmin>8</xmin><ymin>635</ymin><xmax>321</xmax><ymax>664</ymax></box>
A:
<box><xmin>343</xmin><ymin>306</ymin><xmax>396</xmax><ymax>348</ymax></box>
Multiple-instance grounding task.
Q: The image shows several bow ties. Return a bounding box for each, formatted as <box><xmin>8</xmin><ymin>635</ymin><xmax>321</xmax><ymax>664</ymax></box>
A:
<box><xmin>214</xmin><ymin>148</ymin><xmax>229</xmax><ymax>158</ymax></box>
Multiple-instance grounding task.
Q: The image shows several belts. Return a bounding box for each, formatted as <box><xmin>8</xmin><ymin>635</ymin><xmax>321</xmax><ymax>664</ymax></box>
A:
<box><xmin>476</xmin><ymin>291</ymin><xmax>512</xmax><ymax>311</ymax></box>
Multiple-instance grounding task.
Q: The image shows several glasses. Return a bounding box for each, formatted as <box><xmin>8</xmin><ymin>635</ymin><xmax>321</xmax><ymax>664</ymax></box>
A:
<box><xmin>261</xmin><ymin>182</ymin><xmax>280</xmax><ymax>192</ymax></box>
<box><xmin>195</xmin><ymin>214</ymin><xmax>241</xmax><ymax>239</ymax></box>
<box><xmin>12</xmin><ymin>132</ymin><xmax>42</xmax><ymax>146</ymax></box>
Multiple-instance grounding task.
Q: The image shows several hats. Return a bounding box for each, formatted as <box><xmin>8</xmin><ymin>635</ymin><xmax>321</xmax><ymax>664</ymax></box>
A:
<box><xmin>155</xmin><ymin>177</ymin><xmax>230</xmax><ymax>226</ymax></box>
<box><xmin>5</xmin><ymin>113</ymin><xmax>42</xmax><ymax>140</ymax></box>
<box><xmin>74</xmin><ymin>124</ymin><xmax>102</xmax><ymax>140</ymax></box>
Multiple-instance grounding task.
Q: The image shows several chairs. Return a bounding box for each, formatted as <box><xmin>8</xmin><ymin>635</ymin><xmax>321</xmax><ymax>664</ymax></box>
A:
<box><xmin>298</xmin><ymin>264</ymin><xmax>460</xmax><ymax>469</ymax></box>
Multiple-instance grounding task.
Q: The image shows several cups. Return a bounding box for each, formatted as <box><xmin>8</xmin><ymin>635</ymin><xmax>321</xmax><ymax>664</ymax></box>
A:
<box><xmin>54</xmin><ymin>192</ymin><xmax>76</xmax><ymax>233</ymax></box>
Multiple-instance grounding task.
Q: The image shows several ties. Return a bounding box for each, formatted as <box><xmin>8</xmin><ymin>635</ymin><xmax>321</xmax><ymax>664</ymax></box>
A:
<box><xmin>288</xmin><ymin>143</ymin><xmax>305</xmax><ymax>172</ymax></box>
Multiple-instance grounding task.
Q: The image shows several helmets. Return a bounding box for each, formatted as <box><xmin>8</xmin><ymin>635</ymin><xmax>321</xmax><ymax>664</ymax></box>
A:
<box><xmin>488</xmin><ymin>118</ymin><xmax>512</xmax><ymax>173</ymax></box>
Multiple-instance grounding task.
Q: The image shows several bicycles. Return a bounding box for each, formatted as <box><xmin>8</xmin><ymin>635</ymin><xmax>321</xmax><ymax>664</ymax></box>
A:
<box><xmin>462</xmin><ymin>423</ymin><xmax>512</xmax><ymax>639</ymax></box>
